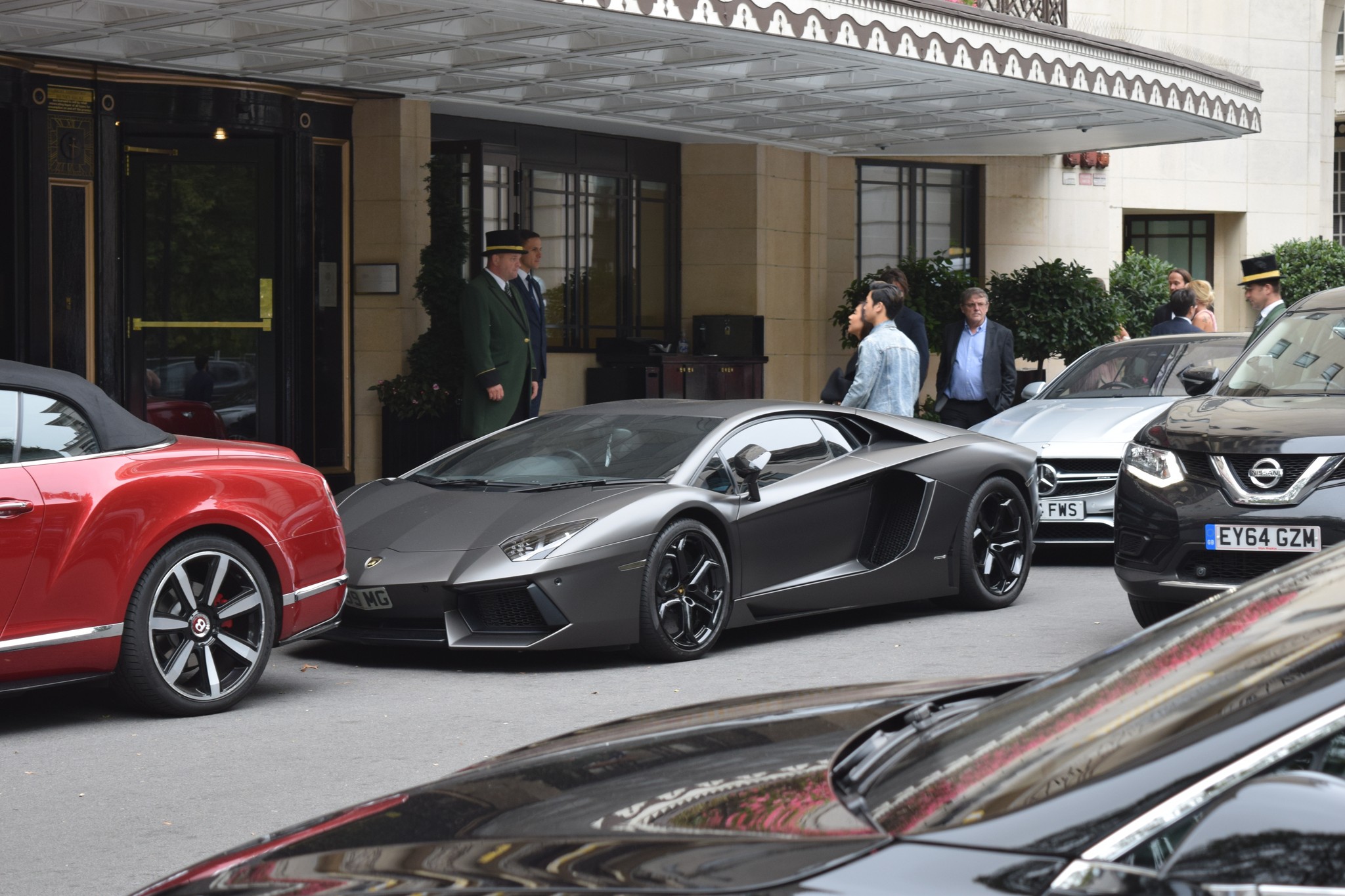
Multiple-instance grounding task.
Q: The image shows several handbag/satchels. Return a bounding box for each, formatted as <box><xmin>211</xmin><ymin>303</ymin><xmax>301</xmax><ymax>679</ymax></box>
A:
<box><xmin>821</xmin><ymin>366</ymin><xmax>853</xmax><ymax>401</ymax></box>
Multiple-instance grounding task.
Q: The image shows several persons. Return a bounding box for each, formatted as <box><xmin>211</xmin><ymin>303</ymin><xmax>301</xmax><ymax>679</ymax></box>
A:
<box><xmin>843</xmin><ymin>301</ymin><xmax>874</xmax><ymax>382</ymax></box>
<box><xmin>1113</xmin><ymin>324</ymin><xmax>1131</xmax><ymax>378</ymax></box>
<box><xmin>1153</xmin><ymin>268</ymin><xmax>1191</xmax><ymax>326</ymax></box>
<box><xmin>1237</xmin><ymin>254</ymin><xmax>1301</xmax><ymax>395</ymax></box>
<box><xmin>146</xmin><ymin>369</ymin><xmax>161</xmax><ymax>394</ymax></box>
<box><xmin>185</xmin><ymin>353</ymin><xmax>214</xmax><ymax>403</ymax></box>
<box><xmin>508</xmin><ymin>230</ymin><xmax>547</xmax><ymax>416</ymax></box>
<box><xmin>1187</xmin><ymin>280</ymin><xmax>1218</xmax><ymax>333</ymax></box>
<box><xmin>1147</xmin><ymin>288</ymin><xmax>1206</xmax><ymax>387</ymax></box>
<box><xmin>880</xmin><ymin>268</ymin><xmax>929</xmax><ymax>418</ymax></box>
<box><xmin>840</xmin><ymin>280</ymin><xmax>920</xmax><ymax>418</ymax></box>
<box><xmin>460</xmin><ymin>230</ymin><xmax>538</xmax><ymax>441</ymax></box>
<box><xmin>935</xmin><ymin>287</ymin><xmax>1017</xmax><ymax>430</ymax></box>
<box><xmin>1070</xmin><ymin>359</ymin><xmax>1122</xmax><ymax>394</ymax></box>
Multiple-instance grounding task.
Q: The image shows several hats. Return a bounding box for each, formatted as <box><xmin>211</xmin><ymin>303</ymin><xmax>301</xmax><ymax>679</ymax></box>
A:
<box><xmin>480</xmin><ymin>229</ymin><xmax>528</xmax><ymax>256</ymax></box>
<box><xmin>1236</xmin><ymin>254</ymin><xmax>1287</xmax><ymax>285</ymax></box>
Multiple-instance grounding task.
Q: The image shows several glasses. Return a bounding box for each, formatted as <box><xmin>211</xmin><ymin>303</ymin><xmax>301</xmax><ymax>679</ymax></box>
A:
<box><xmin>962</xmin><ymin>302</ymin><xmax>987</xmax><ymax>308</ymax></box>
<box><xmin>1187</xmin><ymin>302</ymin><xmax>1198</xmax><ymax>308</ymax></box>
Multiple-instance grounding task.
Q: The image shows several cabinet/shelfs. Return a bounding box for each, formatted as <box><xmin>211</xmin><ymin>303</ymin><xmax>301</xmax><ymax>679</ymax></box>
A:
<box><xmin>595</xmin><ymin>353</ymin><xmax>770</xmax><ymax>399</ymax></box>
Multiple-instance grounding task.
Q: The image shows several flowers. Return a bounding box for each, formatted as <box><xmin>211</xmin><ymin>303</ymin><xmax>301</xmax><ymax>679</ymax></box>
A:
<box><xmin>368</xmin><ymin>374</ymin><xmax>458</xmax><ymax>412</ymax></box>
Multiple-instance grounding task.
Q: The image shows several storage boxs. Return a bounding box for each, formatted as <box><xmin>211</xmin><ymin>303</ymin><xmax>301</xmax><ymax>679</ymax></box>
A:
<box><xmin>690</xmin><ymin>313</ymin><xmax>765</xmax><ymax>355</ymax></box>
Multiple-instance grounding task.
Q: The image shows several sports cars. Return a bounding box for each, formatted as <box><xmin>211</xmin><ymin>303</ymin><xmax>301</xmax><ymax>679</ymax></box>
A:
<box><xmin>305</xmin><ymin>398</ymin><xmax>1038</xmax><ymax>662</ymax></box>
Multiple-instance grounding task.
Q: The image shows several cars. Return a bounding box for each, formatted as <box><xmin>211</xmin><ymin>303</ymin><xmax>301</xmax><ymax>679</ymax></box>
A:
<box><xmin>0</xmin><ymin>358</ymin><xmax>351</xmax><ymax>718</ymax></box>
<box><xmin>123</xmin><ymin>537</ymin><xmax>1345</xmax><ymax>896</ymax></box>
<box><xmin>145</xmin><ymin>359</ymin><xmax>258</xmax><ymax>441</ymax></box>
<box><xmin>967</xmin><ymin>332</ymin><xmax>1252</xmax><ymax>544</ymax></box>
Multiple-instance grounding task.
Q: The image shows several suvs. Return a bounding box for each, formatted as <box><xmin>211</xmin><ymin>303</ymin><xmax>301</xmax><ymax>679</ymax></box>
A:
<box><xmin>1112</xmin><ymin>286</ymin><xmax>1345</xmax><ymax>629</ymax></box>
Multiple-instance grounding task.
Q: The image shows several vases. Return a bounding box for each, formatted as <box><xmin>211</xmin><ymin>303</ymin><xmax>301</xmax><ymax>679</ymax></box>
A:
<box><xmin>380</xmin><ymin>412</ymin><xmax>461</xmax><ymax>477</ymax></box>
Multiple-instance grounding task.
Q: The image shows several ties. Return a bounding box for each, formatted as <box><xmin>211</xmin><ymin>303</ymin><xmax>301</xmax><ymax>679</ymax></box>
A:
<box><xmin>526</xmin><ymin>274</ymin><xmax>535</xmax><ymax>300</ymax></box>
<box><xmin>505</xmin><ymin>282</ymin><xmax>512</xmax><ymax>298</ymax></box>
<box><xmin>1253</xmin><ymin>313</ymin><xmax>1262</xmax><ymax>332</ymax></box>
